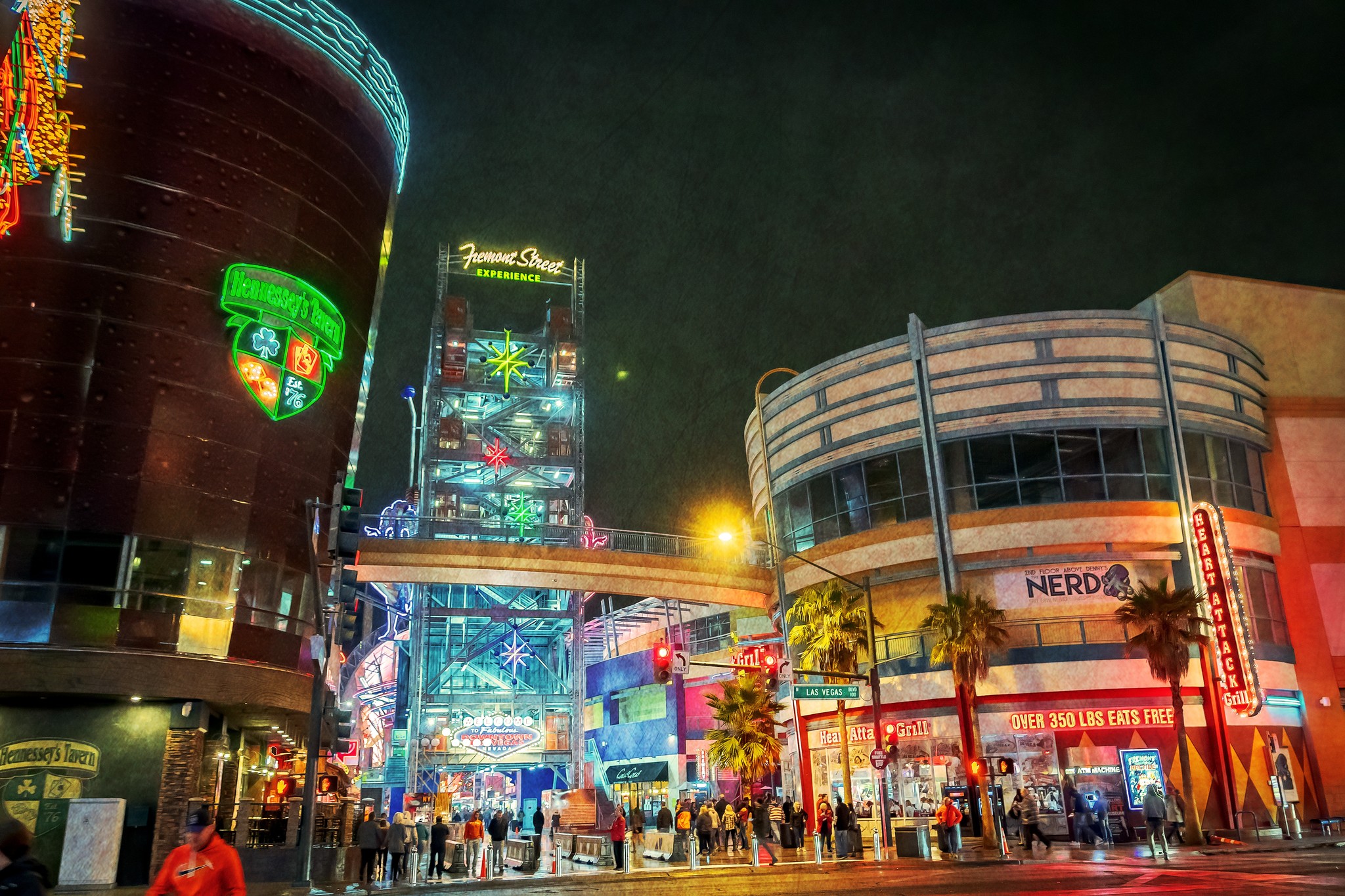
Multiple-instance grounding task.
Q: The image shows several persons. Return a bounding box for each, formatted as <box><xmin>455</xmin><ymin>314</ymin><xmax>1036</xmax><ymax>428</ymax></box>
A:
<box><xmin>676</xmin><ymin>793</ymin><xmax>942</xmax><ymax>866</ymax></box>
<box><xmin>551</xmin><ymin>810</ymin><xmax>561</xmax><ymax>833</ymax></box>
<box><xmin>517</xmin><ymin>807</ymin><xmax>525</xmax><ymax>832</ymax></box>
<box><xmin>0</xmin><ymin>817</ymin><xmax>54</xmax><ymax>896</ymax></box>
<box><xmin>1067</xmin><ymin>784</ymin><xmax>1185</xmax><ymax>861</ymax></box>
<box><xmin>1012</xmin><ymin>789</ymin><xmax>1041</xmax><ymax>846</ymax></box>
<box><xmin>617</xmin><ymin>804</ymin><xmax>620</xmax><ymax>807</ymax></box>
<box><xmin>357</xmin><ymin>811</ymin><xmax>379</xmax><ymax>883</ymax></box>
<box><xmin>488</xmin><ymin>810</ymin><xmax>508</xmax><ymax>871</ymax></box>
<box><xmin>1013</xmin><ymin>789</ymin><xmax>1053</xmax><ymax>850</ymax></box>
<box><xmin>143</xmin><ymin>809</ymin><xmax>249</xmax><ymax>896</ymax></box>
<box><xmin>533</xmin><ymin>807</ymin><xmax>544</xmax><ymax>842</ymax></box>
<box><xmin>375</xmin><ymin>812</ymin><xmax>391</xmax><ymax>827</ymax></box>
<box><xmin>428</xmin><ymin>816</ymin><xmax>450</xmax><ymax>878</ymax></box>
<box><xmin>464</xmin><ymin>811</ymin><xmax>484</xmax><ymax>872</ymax></box>
<box><xmin>936</xmin><ymin>797</ymin><xmax>962</xmax><ymax>859</ymax></box>
<box><xmin>454</xmin><ymin>806</ymin><xmax>515</xmax><ymax>830</ymax></box>
<box><xmin>414</xmin><ymin>822</ymin><xmax>429</xmax><ymax>871</ymax></box>
<box><xmin>398</xmin><ymin>811</ymin><xmax>418</xmax><ymax>874</ymax></box>
<box><xmin>608</xmin><ymin>807</ymin><xmax>626</xmax><ymax>871</ymax></box>
<box><xmin>657</xmin><ymin>801</ymin><xmax>674</xmax><ymax>833</ymax></box>
<box><xmin>381</xmin><ymin>812</ymin><xmax>406</xmax><ymax>882</ymax></box>
<box><xmin>630</xmin><ymin>808</ymin><xmax>646</xmax><ymax>853</ymax></box>
<box><xmin>377</xmin><ymin>820</ymin><xmax>388</xmax><ymax>873</ymax></box>
<box><xmin>620</xmin><ymin>803</ymin><xmax>628</xmax><ymax>828</ymax></box>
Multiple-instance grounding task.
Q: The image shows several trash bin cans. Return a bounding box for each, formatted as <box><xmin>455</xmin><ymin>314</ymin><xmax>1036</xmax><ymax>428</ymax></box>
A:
<box><xmin>894</xmin><ymin>824</ymin><xmax>932</xmax><ymax>858</ymax></box>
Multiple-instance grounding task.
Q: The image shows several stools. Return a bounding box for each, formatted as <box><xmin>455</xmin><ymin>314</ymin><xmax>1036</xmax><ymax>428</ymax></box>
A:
<box><xmin>250</xmin><ymin>828</ymin><xmax>268</xmax><ymax>847</ymax></box>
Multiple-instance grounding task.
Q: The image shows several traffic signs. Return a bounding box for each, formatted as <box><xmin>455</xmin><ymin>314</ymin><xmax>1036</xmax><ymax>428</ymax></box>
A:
<box><xmin>777</xmin><ymin>658</ymin><xmax>794</xmax><ymax>682</ymax></box>
<box><xmin>671</xmin><ymin>650</ymin><xmax>690</xmax><ymax>674</ymax></box>
<box><xmin>790</xmin><ymin>684</ymin><xmax>860</xmax><ymax>700</ymax></box>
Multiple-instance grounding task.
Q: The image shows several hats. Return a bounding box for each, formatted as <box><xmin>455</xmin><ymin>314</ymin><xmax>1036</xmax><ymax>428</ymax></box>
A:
<box><xmin>183</xmin><ymin>809</ymin><xmax>215</xmax><ymax>834</ymax></box>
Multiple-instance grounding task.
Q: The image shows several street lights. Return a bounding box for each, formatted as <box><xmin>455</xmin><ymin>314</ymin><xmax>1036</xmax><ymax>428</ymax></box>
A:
<box><xmin>718</xmin><ymin>532</ymin><xmax>894</xmax><ymax>848</ymax></box>
<box><xmin>401</xmin><ymin>386</ymin><xmax>417</xmax><ymax>487</ymax></box>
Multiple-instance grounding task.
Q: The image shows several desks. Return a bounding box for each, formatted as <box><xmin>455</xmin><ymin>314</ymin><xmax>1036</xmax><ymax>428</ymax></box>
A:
<box><xmin>231</xmin><ymin>816</ymin><xmax>288</xmax><ymax>847</ymax></box>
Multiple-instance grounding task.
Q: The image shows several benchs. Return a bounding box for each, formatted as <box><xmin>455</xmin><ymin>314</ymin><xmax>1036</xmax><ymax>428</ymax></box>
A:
<box><xmin>1309</xmin><ymin>817</ymin><xmax>1345</xmax><ymax>836</ymax></box>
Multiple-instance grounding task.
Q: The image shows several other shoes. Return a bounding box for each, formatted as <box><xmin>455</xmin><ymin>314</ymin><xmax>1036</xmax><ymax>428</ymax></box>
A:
<box><xmin>356</xmin><ymin>828</ymin><xmax>1112</xmax><ymax>885</ymax></box>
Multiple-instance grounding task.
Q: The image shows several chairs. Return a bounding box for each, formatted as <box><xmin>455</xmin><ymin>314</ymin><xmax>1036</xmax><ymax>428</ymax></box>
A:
<box><xmin>314</xmin><ymin>816</ymin><xmax>333</xmax><ymax>845</ymax></box>
<box><xmin>330</xmin><ymin>818</ymin><xmax>340</xmax><ymax>842</ymax></box>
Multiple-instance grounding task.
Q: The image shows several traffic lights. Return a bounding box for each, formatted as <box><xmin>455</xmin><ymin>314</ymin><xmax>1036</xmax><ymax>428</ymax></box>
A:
<box><xmin>329</xmin><ymin>709</ymin><xmax>352</xmax><ymax>756</ymax></box>
<box><xmin>653</xmin><ymin>643</ymin><xmax>672</xmax><ymax>684</ymax></box>
<box><xmin>1268</xmin><ymin>733</ymin><xmax>1279</xmax><ymax>754</ymax></box>
<box><xmin>760</xmin><ymin>654</ymin><xmax>779</xmax><ymax>692</ymax></box>
<box><xmin>969</xmin><ymin>759</ymin><xmax>988</xmax><ymax>775</ymax></box>
<box><xmin>883</xmin><ymin>723</ymin><xmax>899</xmax><ymax>759</ymax></box>
<box><xmin>327</xmin><ymin>483</ymin><xmax>362</xmax><ymax>644</ymax></box>
<box><xmin>997</xmin><ymin>758</ymin><xmax>1015</xmax><ymax>775</ymax></box>
